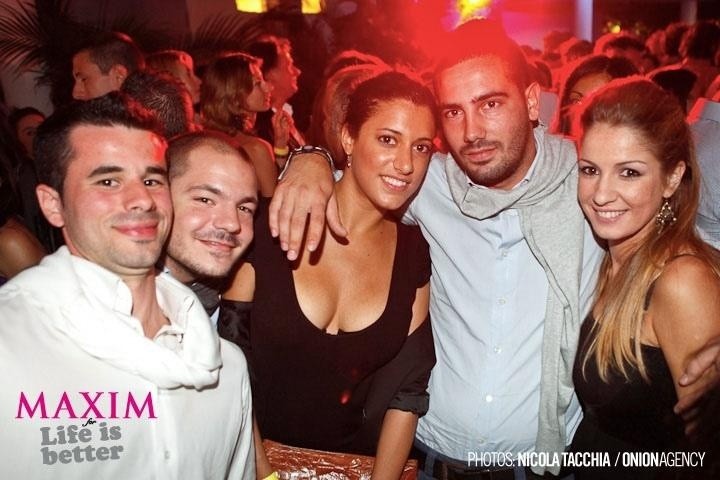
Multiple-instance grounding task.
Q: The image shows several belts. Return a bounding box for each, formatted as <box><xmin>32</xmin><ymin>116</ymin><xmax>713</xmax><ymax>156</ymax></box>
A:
<box><xmin>411</xmin><ymin>447</ymin><xmax>543</xmax><ymax>480</ymax></box>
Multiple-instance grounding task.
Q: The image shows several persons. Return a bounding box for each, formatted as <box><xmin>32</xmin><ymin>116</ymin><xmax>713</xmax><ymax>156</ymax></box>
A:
<box><xmin>1</xmin><ymin>0</ymin><xmax>720</xmax><ymax>480</ymax></box>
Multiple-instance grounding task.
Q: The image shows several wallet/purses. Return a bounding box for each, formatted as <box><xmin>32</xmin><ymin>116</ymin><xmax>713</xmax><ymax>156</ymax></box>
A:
<box><xmin>261</xmin><ymin>438</ymin><xmax>418</xmax><ymax>480</ymax></box>
